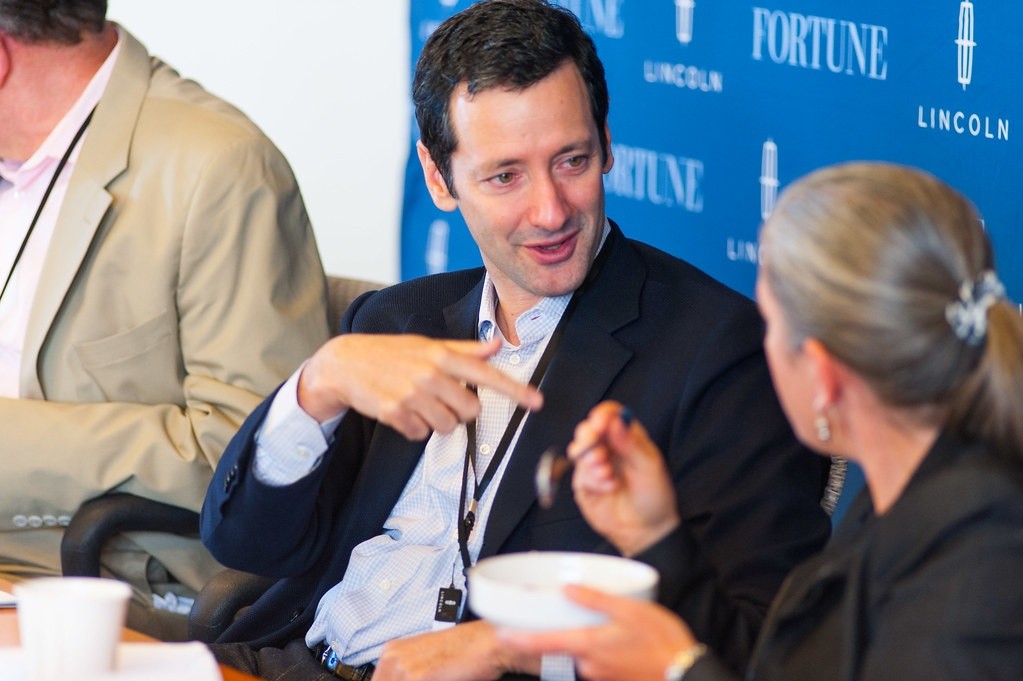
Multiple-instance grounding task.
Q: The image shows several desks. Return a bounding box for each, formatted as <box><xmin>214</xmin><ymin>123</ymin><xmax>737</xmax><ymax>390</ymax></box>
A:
<box><xmin>0</xmin><ymin>577</ymin><xmax>269</xmax><ymax>681</ymax></box>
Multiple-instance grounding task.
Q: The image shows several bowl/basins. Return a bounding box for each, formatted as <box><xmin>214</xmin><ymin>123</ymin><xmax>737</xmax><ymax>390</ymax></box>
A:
<box><xmin>468</xmin><ymin>548</ymin><xmax>660</xmax><ymax>634</ymax></box>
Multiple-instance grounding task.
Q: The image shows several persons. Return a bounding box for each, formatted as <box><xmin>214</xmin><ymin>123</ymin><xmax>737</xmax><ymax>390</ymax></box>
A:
<box><xmin>0</xmin><ymin>0</ymin><xmax>331</xmax><ymax>602</ymax></box>
<box><xmin>188</xmin><ymin>0</ymin><xmax>830</xmax><ymax>679</ymax></box>
<box><xmin>513</xmin><ymin>157</ymin><xmax>1023</xmax><ymax>681</ymax></box>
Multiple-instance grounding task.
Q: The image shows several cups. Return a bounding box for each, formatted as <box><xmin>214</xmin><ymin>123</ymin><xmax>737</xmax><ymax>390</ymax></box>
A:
<box><xmin>14</xmin><ymin>575</ymin><xmax>134</xmax><ymax>674</ymax></box>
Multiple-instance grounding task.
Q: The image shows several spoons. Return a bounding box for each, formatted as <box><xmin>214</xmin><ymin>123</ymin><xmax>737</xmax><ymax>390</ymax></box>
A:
<box><xmin>533</xmin><ymin>405</ymin><xmax>635</xmax><ymax>509</ymax></box>
